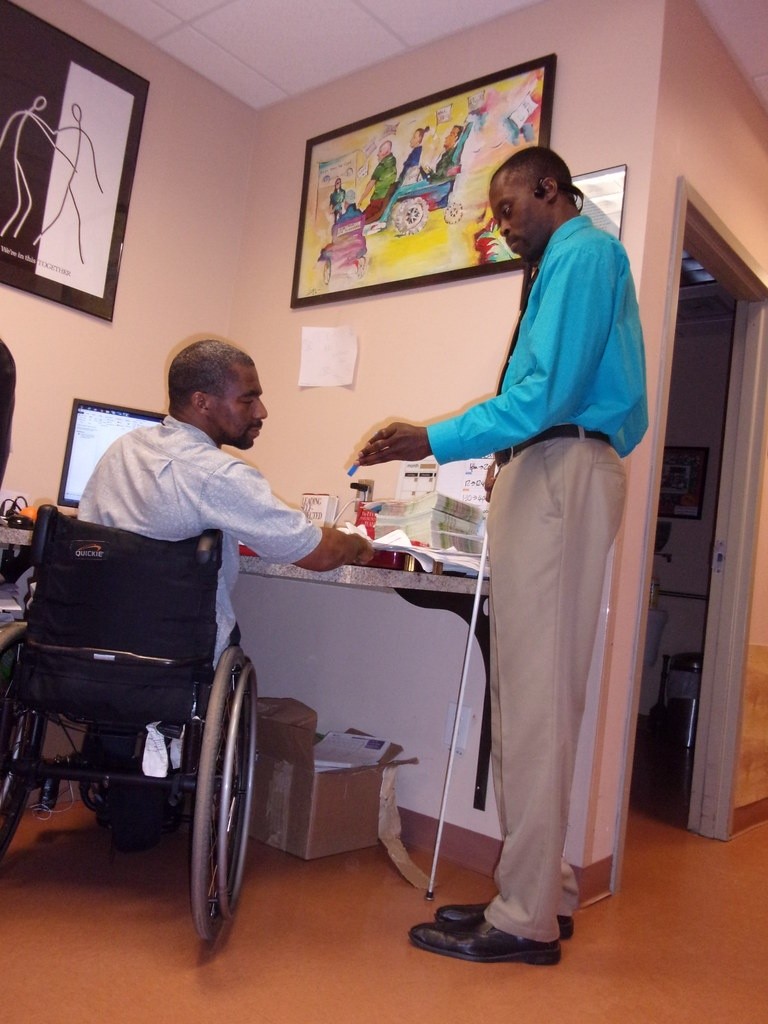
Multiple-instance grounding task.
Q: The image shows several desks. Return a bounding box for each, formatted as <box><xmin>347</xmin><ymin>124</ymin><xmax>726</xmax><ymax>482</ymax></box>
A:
<box><xmin>0</xmin><ymin>527</ymin><xmax>491</xmax><ymax>812</ymax></box>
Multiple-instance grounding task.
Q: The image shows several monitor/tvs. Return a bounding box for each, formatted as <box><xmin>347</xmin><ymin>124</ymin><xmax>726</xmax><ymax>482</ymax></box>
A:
<box><xmin>57</xmin><ymin>396</ymin><xmax>169</xmax><ymax>506</ymax></box>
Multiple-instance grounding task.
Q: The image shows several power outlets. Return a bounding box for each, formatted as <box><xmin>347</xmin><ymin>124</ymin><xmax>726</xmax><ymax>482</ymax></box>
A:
<box><xmin>443</xmin><ymin>701</ymin><xmax>473</xmax><ymax>750</ymax></box>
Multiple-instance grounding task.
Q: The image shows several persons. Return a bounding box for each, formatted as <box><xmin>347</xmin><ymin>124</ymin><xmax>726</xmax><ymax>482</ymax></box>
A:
<box><xmin>78</xmin><ymin>339</ymin><xmax>378</xmax><ymax>671</ymax></box>
<box><xmin>355</xmin><ymin>147</ymin><xmax>647</xmax><ymax>967</ymax></box>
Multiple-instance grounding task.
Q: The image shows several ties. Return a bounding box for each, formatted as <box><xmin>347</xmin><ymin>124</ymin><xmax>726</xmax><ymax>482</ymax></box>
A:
<box><xmin>496</xmin><ymin>266</ymin><xmax>541</xmax><ymax>396</ymax></box>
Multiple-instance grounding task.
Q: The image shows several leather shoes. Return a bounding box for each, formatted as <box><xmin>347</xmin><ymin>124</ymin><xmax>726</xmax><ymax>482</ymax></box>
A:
<box><xmin>434</xmin><ymin>902</ymin><xmax>573</xmax><ymax>941</ymax></box>
<box><xmin>408</xmin><ymin>916</ymin><xmax>561</xmax><ymax>965</ymax></box>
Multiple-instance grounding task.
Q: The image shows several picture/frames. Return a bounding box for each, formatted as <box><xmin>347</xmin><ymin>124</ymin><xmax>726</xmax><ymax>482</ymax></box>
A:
<box><xmin>657</xmin><ymin>444</ymin><xmax>711</xmax><ymax>520</ymax></box>
<box><xmin>288</xmin><ymin>51</ymin><xmax>557</xmax><ymax>309</ymax></box>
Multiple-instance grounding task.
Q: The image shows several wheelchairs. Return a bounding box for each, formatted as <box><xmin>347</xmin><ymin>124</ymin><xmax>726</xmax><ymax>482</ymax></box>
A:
<box><xmin>0</xmin><ymin>503</ymin><xmax>259</xmax><ymax>948</ymax></box>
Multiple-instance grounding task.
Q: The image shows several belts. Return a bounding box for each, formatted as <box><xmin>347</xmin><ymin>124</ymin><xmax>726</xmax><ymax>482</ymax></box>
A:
<box><xmin>494</xmin><ymin>424</ymin><xmax>610</xmax><ymax>468</ymax></box>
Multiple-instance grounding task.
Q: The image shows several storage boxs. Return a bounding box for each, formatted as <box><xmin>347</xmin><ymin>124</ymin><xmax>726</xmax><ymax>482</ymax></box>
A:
<box><xmin>248</xmin><ymin>697</ymin><xmax>418</xmax><ymax>861</ymax></box>
